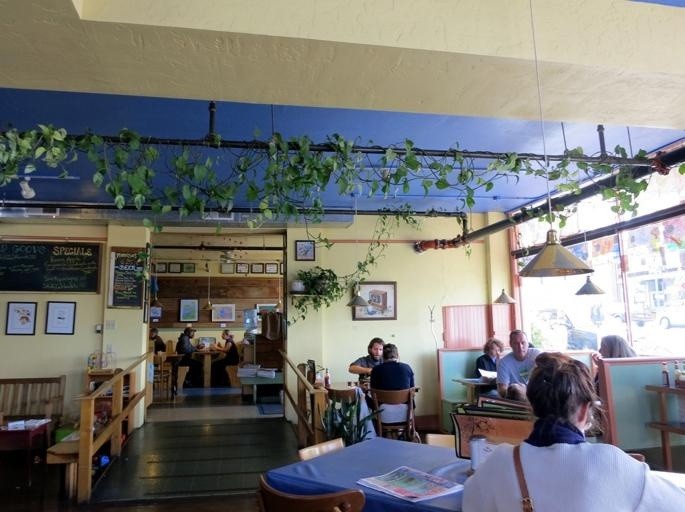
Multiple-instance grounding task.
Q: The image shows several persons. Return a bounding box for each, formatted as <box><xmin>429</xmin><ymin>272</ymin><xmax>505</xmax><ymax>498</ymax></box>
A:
<box><xmin>349</xmin><ymin>338</ymin><xmax>384</xmax><ymax>431</ymax></box>
<box><xmin>175</xmin><ymin>326</ymin><xmax>206</xmax><ymax>388</ymax></box>
<box><xmin>509</xmin><ymin>382</ymin><xmax>527</xmax><ymax>399</ymax></box>
<box><xmin>208</xmin><ymin>329</ymin><xmax>239</xmax><ymax>387</ymax></box>
<box><xmin>149</xmin><ymin>328</ymin><xmax>166</xmax><ymax>370</ymax></box>
<box><xmin>495</xmin><ymin>331</ymin><xmax>543</xmax><ymax>400</ymax></box>
<box><xmin>590</xmin><ymin>336</ymin><xmax>636</xmax><ymax>399</ymax></box>
<box><xmin>370</xmin><ymin>343</ymin><xmax>416</xmax><ymax>438</ymax></box>
<box><xmin>460</xmin><ymin>353</ymin><xmax>684</xmax><ymax>512</ymax></box>
<box><xmin>475</xmin><ymin>339</ymin><xmax>505</xmax><ymax>401</ymax></box>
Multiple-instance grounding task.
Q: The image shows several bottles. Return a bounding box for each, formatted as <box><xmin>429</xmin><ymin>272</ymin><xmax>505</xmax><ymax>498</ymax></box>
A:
<box><xmin>325</xmin><ymin>369</ymin><xmax>331</xmax><ymax>387</ymax></box>
<box><xmin>469</xmin><ymin>434</ymin><xmax>489</xmax><ymax>470</ymax></box>
<box><xmin>675</xmin><ymin>359</ymin><xmax>685</xmax><ymax>389</ymax></box>
<box><xmin>662</xmin><ymin>362</ymin><xmax>670</xmax><ymax>387</ymax></box>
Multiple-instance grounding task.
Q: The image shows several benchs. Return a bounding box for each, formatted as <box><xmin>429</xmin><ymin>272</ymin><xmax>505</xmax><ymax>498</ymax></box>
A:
<box><xmin>0</xmin><ymin>376</ymin><xmax>63</xmax><ymax>429</ymax></box>
<box><xmin>148</xmin><ymin>341</ymin><xmax>247</xmax><ymax>403</ymax></box>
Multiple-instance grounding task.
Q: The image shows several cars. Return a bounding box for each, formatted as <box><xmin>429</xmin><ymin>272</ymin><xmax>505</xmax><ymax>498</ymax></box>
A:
<box><xmin>590</xmin><ymin>303</ymin><xmax>653</xmax><ymax>329</ymax></box>
<box><xmin>527</xmin><ymin>308</ymin><xmax>598</xmax><ymax>351</ymax></box>
<box><xmin>656</xmin><ymin>302</ymin><xmax>685</xmax><ymax>330</ymax></box>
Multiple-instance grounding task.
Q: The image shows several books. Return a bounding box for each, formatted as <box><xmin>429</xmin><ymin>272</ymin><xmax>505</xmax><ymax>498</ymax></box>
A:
<box><xmin>121</xmin><ymin>384</ymin><xmax>130</xmax><ymax>398</ymax></box>
<box><xmin>358</xmin><ymin>465</ymin><xmax>464</xmax><ymax>504</ymax></box>
<box><xmin>236</xmin><ymin>361</ymin><xmax>277</xmax><ymax>381</ymax></box>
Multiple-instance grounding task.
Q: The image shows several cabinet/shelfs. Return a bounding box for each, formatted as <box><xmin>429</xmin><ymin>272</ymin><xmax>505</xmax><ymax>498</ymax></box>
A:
<box><xmin>88</xmin><ymin>370</ymin><xmax>135</xmax><ymax>435</ymax></box>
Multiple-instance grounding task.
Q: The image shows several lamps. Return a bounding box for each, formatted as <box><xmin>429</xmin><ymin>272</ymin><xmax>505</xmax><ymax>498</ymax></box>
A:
<box><xmin>494</xmin><ymin>290</ymin><xmax>519</xmax><ymax>307</ymax></box>
<box><xmin>518</xmin><ymin>156</ymin><xmax>593</xmax><ymax>277</ymax></box>
<box><xmin>577</xmin><ymin>222</ymin><xmax>599</xmax><ymax>296</ymax></box>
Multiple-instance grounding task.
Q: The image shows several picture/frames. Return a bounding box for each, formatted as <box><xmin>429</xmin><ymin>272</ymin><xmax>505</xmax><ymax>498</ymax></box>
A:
<box><xmin>353</xmin><ymin>280</ymin><xmax>397</xmax><ymax>321</ymax></box>
<box><xmin>5</xmin><ymin>301</ymin><xmax>37</xmax><ymax>336</ymax></box>
<box><xmin>147</xmin><ymin>261</ymin><xmax>283</xmax><ymax>274</ymax></box>
<box><xmin>46</xmin><ymin>301</ymin><xmax>77</xmax><ymax>335</ymax></box>
<box><xmin>296</xmin><ymin>240</ymin><xmax>315</xmax><ymax>261</ymax></box>
<box><xmin>211</xmin><ymin>303</ymin><xmax>236</xmax><ymax>323</ymax></box>
<box><xmin>255</xmin><ymin>303</ymin><xmax>276</xmax><ymax>321</ymax></box>
<box><xmin>178</xmin><ymin>298</ymin><xmax>199</xmax><ymax>322</ymax></box>
<box><xmin>150</xmin><ymin>306</ymin><xmax>162</xmax><ymax>319</ymax></box>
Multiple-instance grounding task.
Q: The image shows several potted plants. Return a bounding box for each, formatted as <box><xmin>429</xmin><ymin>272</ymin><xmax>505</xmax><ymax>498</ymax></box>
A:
<box><xmin>295</xmin><ymin>268</ymin><xmax>340</xmax><ymax>317</ymax></box>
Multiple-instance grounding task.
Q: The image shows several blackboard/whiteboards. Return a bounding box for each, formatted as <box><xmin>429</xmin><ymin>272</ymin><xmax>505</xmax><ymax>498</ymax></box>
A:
<box><xmin>0</xmin><ymin>240</ymin><xmax>103</xmax><ymax>295</ymax></box>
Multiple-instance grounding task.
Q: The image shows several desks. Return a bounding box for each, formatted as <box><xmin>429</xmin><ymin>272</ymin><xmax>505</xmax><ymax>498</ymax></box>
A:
<box><xmin>0</xmin><ymin>419</ymin><xmax>49</xmax><ymax>512</ymax></box>
